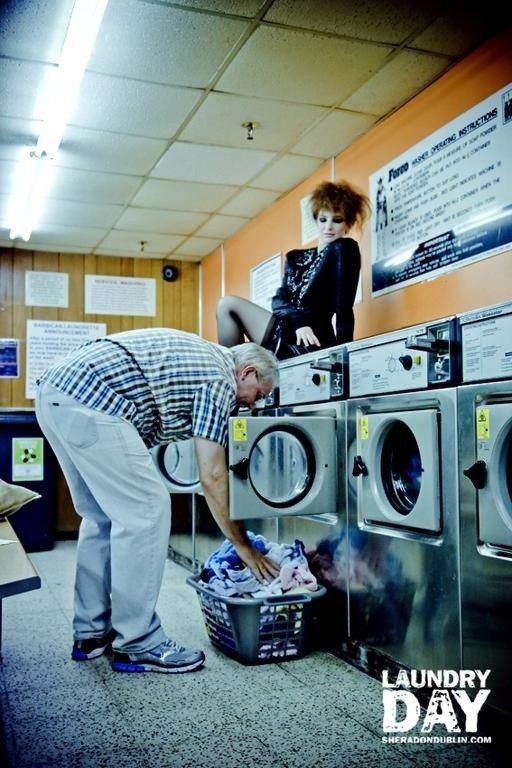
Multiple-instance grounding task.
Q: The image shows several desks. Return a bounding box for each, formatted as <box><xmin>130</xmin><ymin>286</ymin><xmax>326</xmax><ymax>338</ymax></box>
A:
<box><xmin>0</xmin><ymin>511</ymin><xmax>42</xmax><ymax>598</ymax></box>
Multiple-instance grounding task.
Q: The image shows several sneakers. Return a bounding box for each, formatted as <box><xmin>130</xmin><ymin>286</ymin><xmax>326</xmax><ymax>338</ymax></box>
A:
<box><xmin>70</xmin><ymin>636</ymin><xmax>112</xmax><ymax>660</ymax></box>
<box><xmin>112</xmin><ymin>639</ymin><xmax>204</xmax><ymax>674</ymax></box>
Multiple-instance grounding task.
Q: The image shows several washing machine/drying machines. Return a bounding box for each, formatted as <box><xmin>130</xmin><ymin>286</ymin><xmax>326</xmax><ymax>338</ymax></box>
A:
<box><xmin>150</xmin><ymin>302</ymin><xmax>512</xmax><ymax>732</ymax></box>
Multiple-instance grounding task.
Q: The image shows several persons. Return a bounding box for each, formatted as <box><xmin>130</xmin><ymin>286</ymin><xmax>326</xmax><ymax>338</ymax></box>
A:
<box><xmin>32</xmin><ymin>324</ymin><xmax>281</xmax><ymax>678</ymax></box>
<box><xmin>214</xmin><ymin>180</ymin><xmax>374</xmax><ymax>362</ymax></box>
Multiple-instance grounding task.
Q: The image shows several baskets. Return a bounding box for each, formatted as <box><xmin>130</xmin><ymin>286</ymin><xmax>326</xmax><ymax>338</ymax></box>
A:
<box><xmin>186</xmin><ymin>572</ymin><xmax>327</xmax><ymax>665</ymax></box>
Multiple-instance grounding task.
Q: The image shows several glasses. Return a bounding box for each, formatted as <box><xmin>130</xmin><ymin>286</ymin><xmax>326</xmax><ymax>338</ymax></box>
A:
<box><xmin>255</xmin><ymin>375</ymin><xmax>265</xmax><ymax>400</ymax></box>
